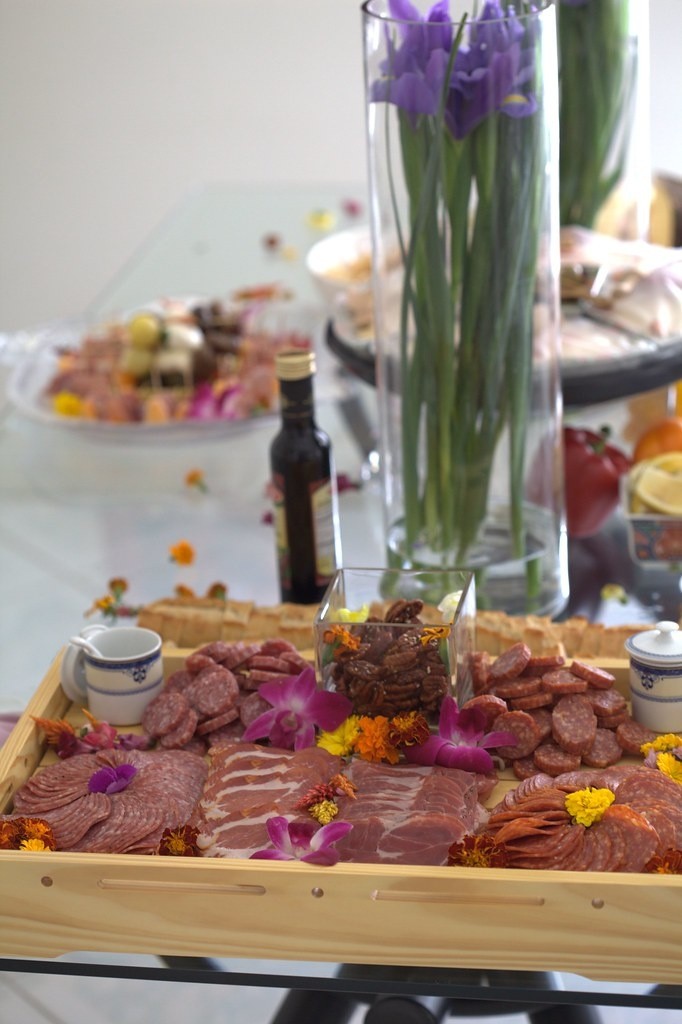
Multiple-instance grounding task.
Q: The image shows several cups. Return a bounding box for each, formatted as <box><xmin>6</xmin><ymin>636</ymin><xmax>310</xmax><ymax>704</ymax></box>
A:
<box><xmin>82</xmin><ymin>626</ymin><xmax>164</xmax><ymax>726</ymax></box>
<box><xmin>313</xmin><ymin>566</ymin><xmax>479</xmax><ymax>728</ymax></box>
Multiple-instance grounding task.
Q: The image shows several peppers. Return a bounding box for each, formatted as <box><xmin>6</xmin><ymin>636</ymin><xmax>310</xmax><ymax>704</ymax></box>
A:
<box><xmin>524</xmin><ymin>425</ymin><xmax>631</xmax><ymax>540</ymax></box>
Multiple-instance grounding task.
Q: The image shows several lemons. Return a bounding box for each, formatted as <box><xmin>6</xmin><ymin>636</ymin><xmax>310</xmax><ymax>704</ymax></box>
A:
<box><xmin>626</xmin><ymin>448</ymin><xmax>682</xmax><ymax>517</ymax></box>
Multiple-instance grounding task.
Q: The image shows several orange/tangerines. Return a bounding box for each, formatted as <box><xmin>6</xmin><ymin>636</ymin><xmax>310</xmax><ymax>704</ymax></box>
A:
<box><xmin>631</xmin><ymin>418</ymin><xmax>682</xmax><ymax>463</ymax></box>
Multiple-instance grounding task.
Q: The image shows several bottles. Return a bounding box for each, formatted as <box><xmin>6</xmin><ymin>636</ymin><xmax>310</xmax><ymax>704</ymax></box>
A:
<box><xmin>268</xmin><ymin>350</ymin><xmax>345</xmax><ymax>605</ymax></box>
<box><xmin>622</xmin><ymin>621</ymin><xmax>682</xmax><ymax>734</ymax></box>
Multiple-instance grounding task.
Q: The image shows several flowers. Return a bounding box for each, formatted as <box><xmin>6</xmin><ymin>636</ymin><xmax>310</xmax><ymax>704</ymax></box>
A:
<box><xmin>361</xmin><ymin>0</ymin><xmax>544</xmax><ymax>156</ymax></box>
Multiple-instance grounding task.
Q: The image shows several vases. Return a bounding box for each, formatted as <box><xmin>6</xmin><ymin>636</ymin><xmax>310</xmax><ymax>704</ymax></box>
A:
<box><xmin>358</xmin><ymin>0</ymin><xmax>560</xmax><ymax>626</ymax></box>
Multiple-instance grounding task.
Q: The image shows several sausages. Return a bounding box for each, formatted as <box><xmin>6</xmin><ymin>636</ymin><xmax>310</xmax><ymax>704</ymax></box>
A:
<box><xmin>3</xmin><ymin>637</ymin><xmax>681</xmax><ymax>876</ymax></box>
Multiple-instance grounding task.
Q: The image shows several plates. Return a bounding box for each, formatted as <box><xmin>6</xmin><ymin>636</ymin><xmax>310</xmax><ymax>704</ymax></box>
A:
<box><xmin>7</xmin><ymin>319</ymin><xmax>290</xmax><ymax>440</ymax></box>
